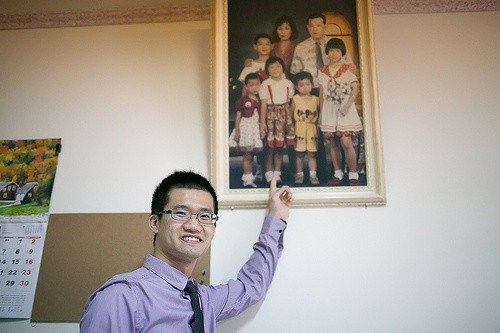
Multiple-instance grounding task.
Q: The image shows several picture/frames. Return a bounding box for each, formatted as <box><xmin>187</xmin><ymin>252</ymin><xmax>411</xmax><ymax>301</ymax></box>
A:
<box><xmin>209</xmin><ymin>0</ymin><xmax>386</xmax><ymax>211</ymax></box>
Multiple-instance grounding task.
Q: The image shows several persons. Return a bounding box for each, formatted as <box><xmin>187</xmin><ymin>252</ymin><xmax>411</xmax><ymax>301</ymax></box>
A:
<box><xmin>257</xmin><ymin>56</ymin><xmax>296</xmax><ymax>183</ymax></box>
<box><xmin>290</xmin><ymin>71</ymin><xmax>321</xmax><ymax>187</ymax></box>
<box><xmin>238</xmin><ymin>33</ymin><xmax>275</xmax><ymax>182</ymax></box>
<box><xmin>79</xmin><ymin>171</ymin><xmax>293</xmax><ymax>333</ymax></box>
<box><xmin>290</xmin><ymin>13</ymin><xmax>357</xmax><ymax>183</ymax></box>
<box><xmin>244</xmin><ymin>16</ymin><xmax>302</xmax><ymax>177</ymax></box>
<box><xmin>318</xmin><ymin>38</ymin><xmax>364</xmax><ymax>186</ymax></box>
<box><xmin>229</xmin><ymin>73</ymin><xmax>263</xmax><ymax>187</ymax></box>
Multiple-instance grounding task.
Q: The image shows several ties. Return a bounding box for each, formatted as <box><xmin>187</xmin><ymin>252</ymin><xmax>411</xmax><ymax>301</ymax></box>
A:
<box><xmin>315</xmin><ymin>41</ymin><xmax>324</xmax><ymax>73</ymax></box>
<box><xmin>184</xmin><ymin>280</ymin><xmax>204</xmax><ymax>332</ymax></box>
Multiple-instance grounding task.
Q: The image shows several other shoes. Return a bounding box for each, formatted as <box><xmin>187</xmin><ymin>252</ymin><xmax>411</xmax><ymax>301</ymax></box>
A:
<box><xmin>267</xmin><ymin>176</ymin><xmax>272</xmax><ymax>183</ymax></box>
<box><xmin>277</xmin><ymin>175</ymin><xmax>283</xmax><ymax>183</ymax></box>
<box><xmin>243</xmin><ymin>180</ymin><xmax>253</xmax><ymax>186</ymax></box>
<box><xmin>255</xmin><ymin>170</ymin><xmax>263</xmax><ymax>182</ymax></box>
<box><xmin>328</xmin><ymin>176</ymin><xmax>343</xmax><ymax>185</ymax></box>
<box><xmin>309</xmin><ymin>176</ymin><xmax>320</xmax><ymax>184</ymax></box>
<box><xmin>350</xmin><ymin>178</ymin><xmax>361</xmax><ymax>185</ymax></box>
<box><xmin>295</xmin><ymin>174</ymin><xmax>304</xmax><ymax>183</ymax></box>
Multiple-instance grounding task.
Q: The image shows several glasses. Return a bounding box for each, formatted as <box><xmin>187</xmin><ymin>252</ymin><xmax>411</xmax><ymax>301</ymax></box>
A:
<box><xmin>157</xmin><ymin>206</ymin><xmax>219</xmax><ymax>223</ymax></box>
